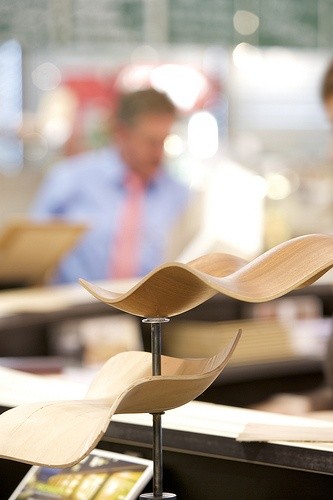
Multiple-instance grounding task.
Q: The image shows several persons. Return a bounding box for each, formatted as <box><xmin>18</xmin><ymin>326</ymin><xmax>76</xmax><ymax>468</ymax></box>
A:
<box><xmin>25</xmin><ymin>89</ymin><xmax>193</xmax><ymax>352</ymax></box>
